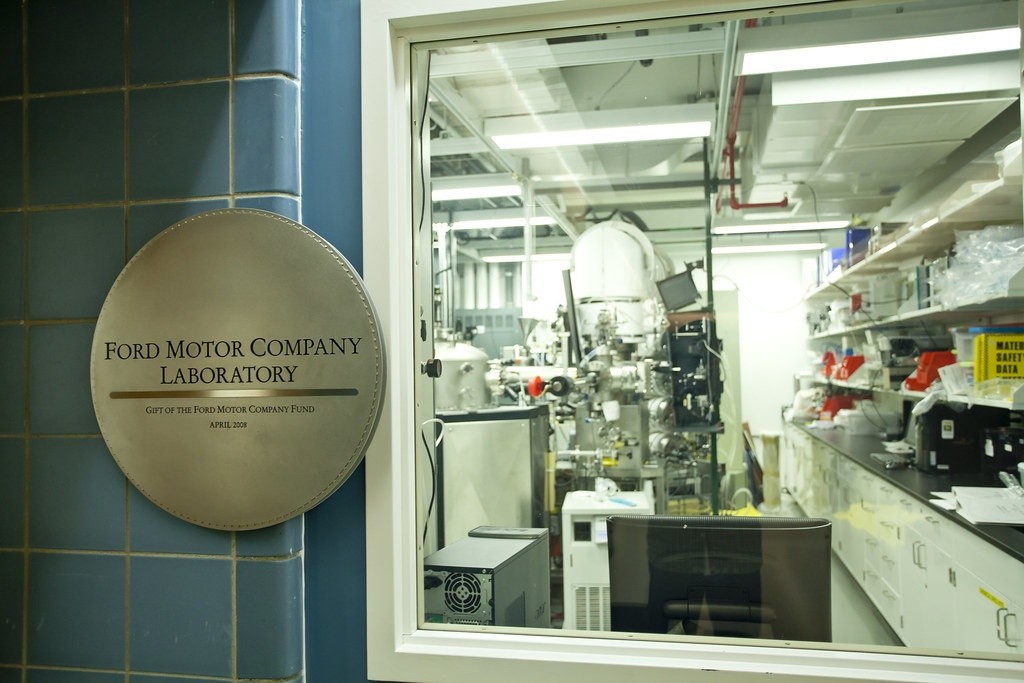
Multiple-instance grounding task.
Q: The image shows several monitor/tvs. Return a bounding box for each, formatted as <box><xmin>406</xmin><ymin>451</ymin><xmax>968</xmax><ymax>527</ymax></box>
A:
<box><xmin>656</xmin><ymin>271</ymin><xmax>701</xmax><ymax>311</ymax></box>
<box><xmin>607</xmin><ymin>516</ymin><xmax>834</xmax><ymax>643</ymax></box>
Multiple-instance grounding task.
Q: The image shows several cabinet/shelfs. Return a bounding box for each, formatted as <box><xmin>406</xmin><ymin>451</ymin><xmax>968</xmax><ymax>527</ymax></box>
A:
<box><xmin>781</xmin><ymin>113</ymin><xmax>1023</xmax><ymax>659</ymax></box>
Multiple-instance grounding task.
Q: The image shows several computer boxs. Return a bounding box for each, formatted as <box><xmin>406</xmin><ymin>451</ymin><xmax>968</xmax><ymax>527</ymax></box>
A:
<box><xmin>424</xmin><ymin>525</ymin><xmax>550</xmax><ymax>628</ymax></box>
